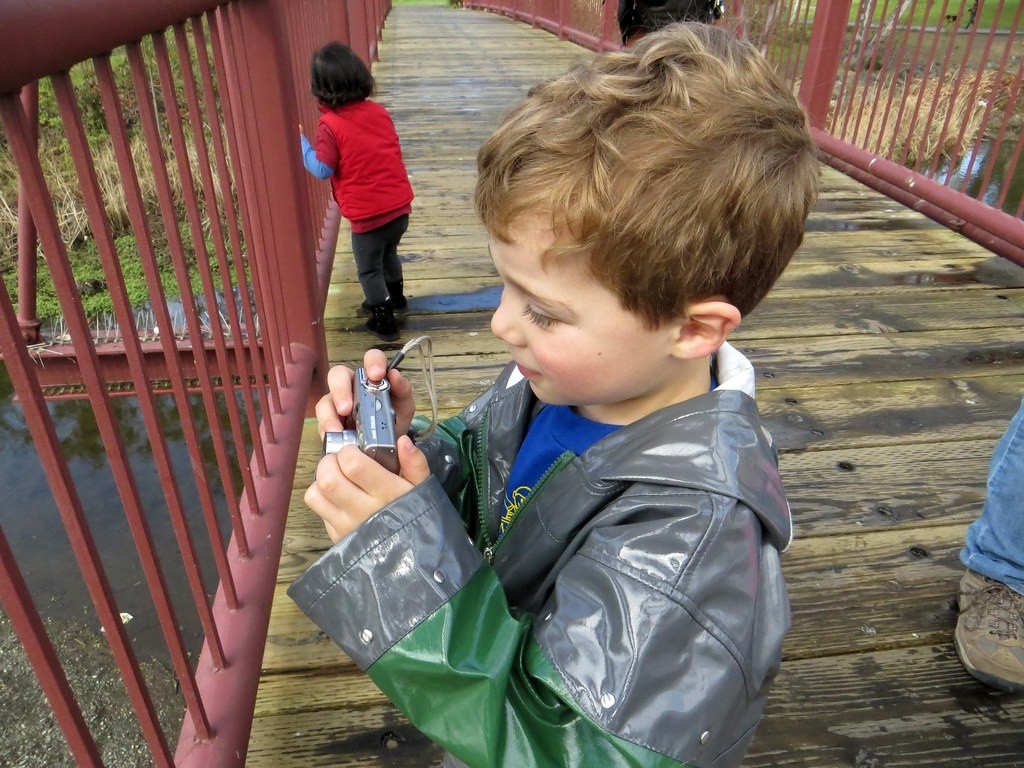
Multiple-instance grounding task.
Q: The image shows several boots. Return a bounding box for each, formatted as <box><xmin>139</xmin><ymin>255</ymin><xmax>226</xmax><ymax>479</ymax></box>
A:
<box><xmin>362</xmin><ymin>278</ymin><xmax>409</xmax><ymax>312</ymax></box>
<box><xmin>365</xmin><ymin>299</ymin><xmax>401</xmax><ymax>341</ymax></box>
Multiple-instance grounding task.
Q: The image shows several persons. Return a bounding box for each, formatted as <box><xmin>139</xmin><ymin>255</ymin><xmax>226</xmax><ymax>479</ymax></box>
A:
<box><xmin>953</xmin><ymin>395</ymin><xmax>1024</xmax><ymax>694</ymax></box>
<box><xmin>298</xmin><ymin>42</ymin><xmax>415</xmax><ymax>345</ymax></box>
<box><xmin>284</xmin><ymin>17</ymin><xmax>824</xmax><ymax>768</ymax></box>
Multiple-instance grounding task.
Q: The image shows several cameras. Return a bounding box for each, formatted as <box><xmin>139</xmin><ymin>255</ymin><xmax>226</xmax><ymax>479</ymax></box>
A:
<box><xmin>324</xmin><ymin>366</ymin><xmax>401</xmax><ymax>477</ymax></box>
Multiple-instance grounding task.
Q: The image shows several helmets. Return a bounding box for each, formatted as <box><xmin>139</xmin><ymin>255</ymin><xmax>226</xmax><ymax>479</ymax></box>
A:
<box><xmin>619</xmin><ymin>0</ymin><xmax>722</xmax><ymax>45</ymax></box>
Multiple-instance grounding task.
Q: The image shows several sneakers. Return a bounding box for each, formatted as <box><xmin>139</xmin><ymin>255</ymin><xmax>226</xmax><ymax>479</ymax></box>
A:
<box><xmin>953</xmin><ymin>567</ymin><xmax>1024</xmax><ymax>693</ymax></box>
